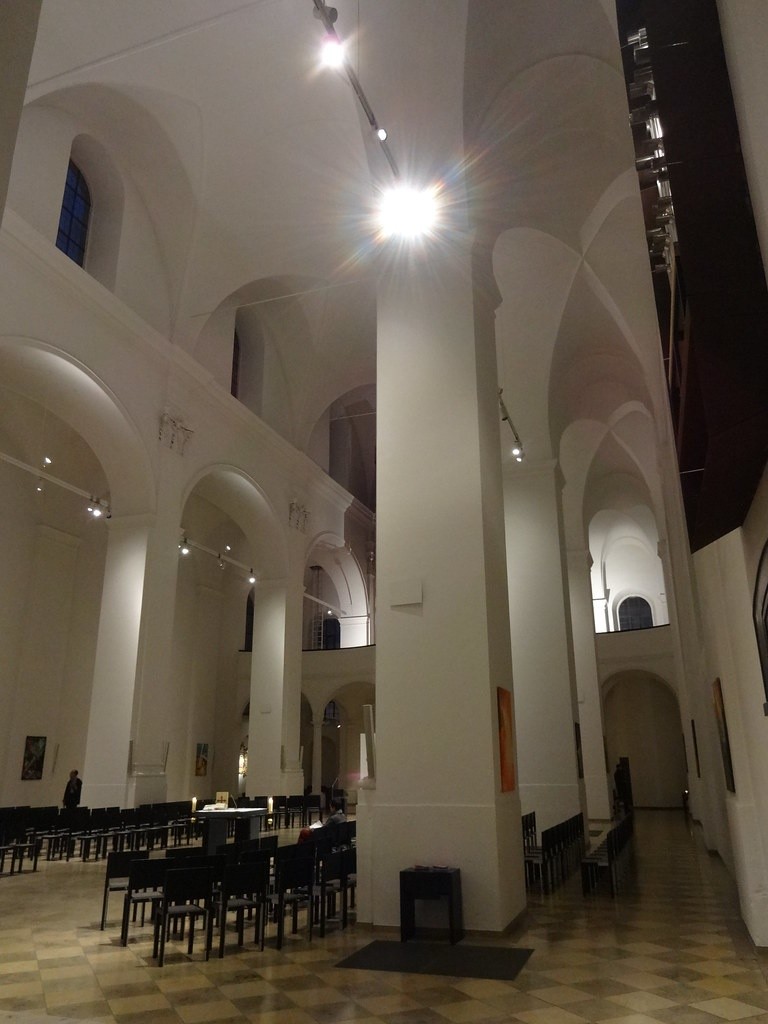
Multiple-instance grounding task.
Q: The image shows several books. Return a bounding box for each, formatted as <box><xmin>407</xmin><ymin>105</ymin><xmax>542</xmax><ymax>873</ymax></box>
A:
<box><xmin>203</xmin><ymin>802</ymin><xmax>227</xmax><ymax>811</ymax></box>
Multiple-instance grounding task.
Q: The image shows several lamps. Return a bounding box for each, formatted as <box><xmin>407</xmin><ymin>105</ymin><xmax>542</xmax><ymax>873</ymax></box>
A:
<box><xmin>500</xmin><ymin>406</ymin><xmax>509</xmax><ymax>421</ymax></box>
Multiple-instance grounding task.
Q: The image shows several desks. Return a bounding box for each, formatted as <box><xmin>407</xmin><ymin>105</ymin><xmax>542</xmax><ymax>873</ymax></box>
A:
<box><xmin>400</xmin><ymin>868</ymin><xmax>465</xmax><ymax>946</ymax></box>
<box><xmin>194</xmin><ymin>808</ymin><xmax>269</xmax><ymax>844</ymax></box>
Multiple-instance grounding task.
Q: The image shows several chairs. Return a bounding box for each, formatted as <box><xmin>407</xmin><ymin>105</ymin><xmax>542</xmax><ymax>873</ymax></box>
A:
<box><xmin>100</xmin><ymin>820</ymin><xmax>357</xmax><ymax>968</ymax></box>
<box><xmin>0</xmin><ymin>789</ymin><xmax>346</xmax><ymax>876</ymax></box>
<box><xmin>522</xmin><ymin>811</ymin><xmax>585</xmax><ymax>895</ymax></box>
<box><xmin>582</xmin><ymin>812</ymin><xmax>634</xmax><ymax>900</ymax></box>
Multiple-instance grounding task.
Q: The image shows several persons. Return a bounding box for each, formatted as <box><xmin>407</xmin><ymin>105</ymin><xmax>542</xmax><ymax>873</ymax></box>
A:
<box><xmin>308</xmin><ymin>798</ymin><xmax>347</xmax><ymax>864</ymax></box>
<box><xmin>61</xmin><ymin>769</ymin><xmax>82</xmax><ymax>809</ymax></box>
